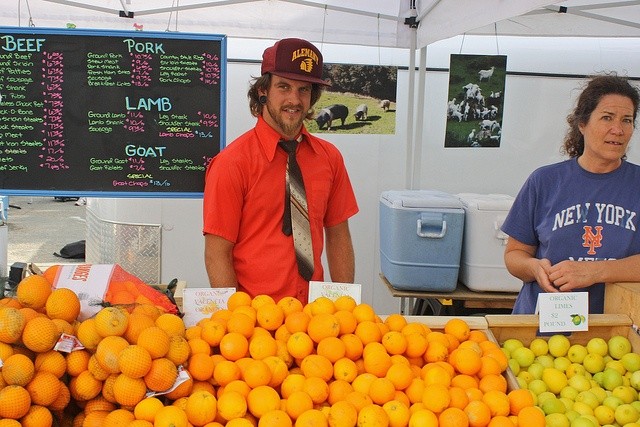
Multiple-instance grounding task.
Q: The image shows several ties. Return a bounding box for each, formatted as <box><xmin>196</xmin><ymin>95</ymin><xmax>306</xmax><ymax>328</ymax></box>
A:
<box><xmin>276</xmin><ymin>134</ymin><xmax>316</xmax><ymax>282</ymax></box>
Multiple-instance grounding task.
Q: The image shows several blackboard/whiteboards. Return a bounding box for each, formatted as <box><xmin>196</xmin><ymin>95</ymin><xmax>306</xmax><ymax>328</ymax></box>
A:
<box><xmin>0</xmin><ymin>26</ymin><xmax>227</xmax><ymax>195</ymax></box>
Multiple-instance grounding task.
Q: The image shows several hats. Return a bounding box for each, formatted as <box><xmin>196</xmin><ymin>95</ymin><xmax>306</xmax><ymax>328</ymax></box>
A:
<box><xmin>261</xmin><ymin>38</ymin><xmax>335</xmax><ymax>91</ymax></box>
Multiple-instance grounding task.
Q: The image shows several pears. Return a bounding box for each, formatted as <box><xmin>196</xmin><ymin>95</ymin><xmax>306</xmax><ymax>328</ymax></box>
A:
<box><xmin>501</xmin><ymin>335</ymin><xmax>640</xmax><ymax>427</ymax></box>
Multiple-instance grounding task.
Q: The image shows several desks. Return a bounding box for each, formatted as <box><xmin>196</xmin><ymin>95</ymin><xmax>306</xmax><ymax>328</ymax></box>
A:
<box><xmin>376</xmin><ymin>273</ymin><xmax>519</xmax><ymax>316</ymax></box>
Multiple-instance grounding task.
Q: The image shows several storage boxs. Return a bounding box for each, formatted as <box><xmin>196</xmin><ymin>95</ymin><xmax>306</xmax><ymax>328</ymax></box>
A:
<box><xmin>379</xmin><ymin>189</ymin><xmax>465</xmax><ymax>293</ymax></box>
<box><xmin>369</xmin><ymin>313</ymin><xmax>526</xmax><ymax>398</ymax></box>
<box><xmin>488</xmin><ymin>312</ymin><xmax>640</xmax><ymax>425</ymax></box>
<box><xmin>457</xmin><ymin>193</ymin><xmax>524</xmax><ymax>294</ymax></box>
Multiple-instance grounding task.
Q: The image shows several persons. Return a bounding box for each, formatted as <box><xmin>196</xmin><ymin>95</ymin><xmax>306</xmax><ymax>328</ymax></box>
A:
<box><xmin>203</xmin><ymin>38</ymin><xmax>360</xmax><ymax>307</ymax></box>
<box><xmin>500</xmin><ymin>70</ymin><xmax>639</xmax><ymax>315</ymax></box>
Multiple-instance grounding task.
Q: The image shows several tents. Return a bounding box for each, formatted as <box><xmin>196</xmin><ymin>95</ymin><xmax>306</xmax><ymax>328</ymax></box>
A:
<box><xmin>0</xmin><ymin>0</ymin><xmax>640</xmax><ymax>191</ymax></box>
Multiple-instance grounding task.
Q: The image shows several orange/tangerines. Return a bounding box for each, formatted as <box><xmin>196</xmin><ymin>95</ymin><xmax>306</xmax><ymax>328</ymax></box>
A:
<box><xmin>1</xmin><ymin>261</ymin><xmax>546</xmax><ymax>427</ymax></box>
